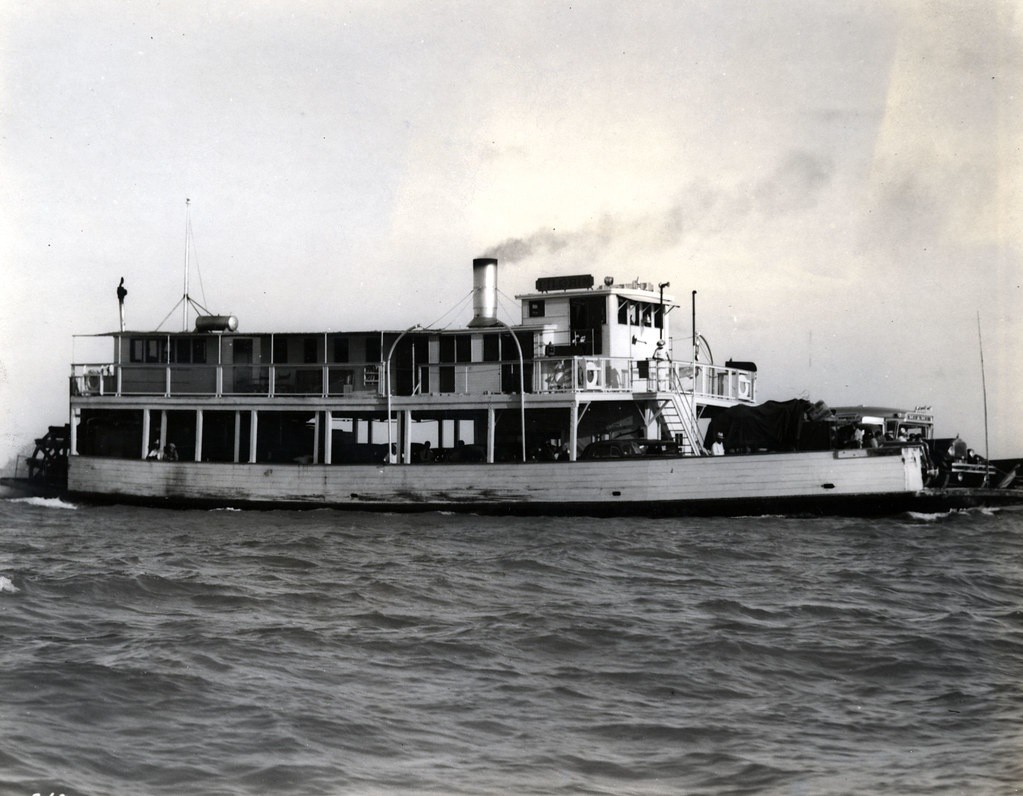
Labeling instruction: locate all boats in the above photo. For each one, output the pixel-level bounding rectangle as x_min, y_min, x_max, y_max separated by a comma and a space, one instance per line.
1, 197, 930, 524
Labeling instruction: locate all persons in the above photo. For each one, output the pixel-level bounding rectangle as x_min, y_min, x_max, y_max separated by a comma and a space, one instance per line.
711, 431, 725, 457
406, 437, 486, 468
819, 418, 1005, 486
146, 438, 162, 461
163, 443, 179, 462
